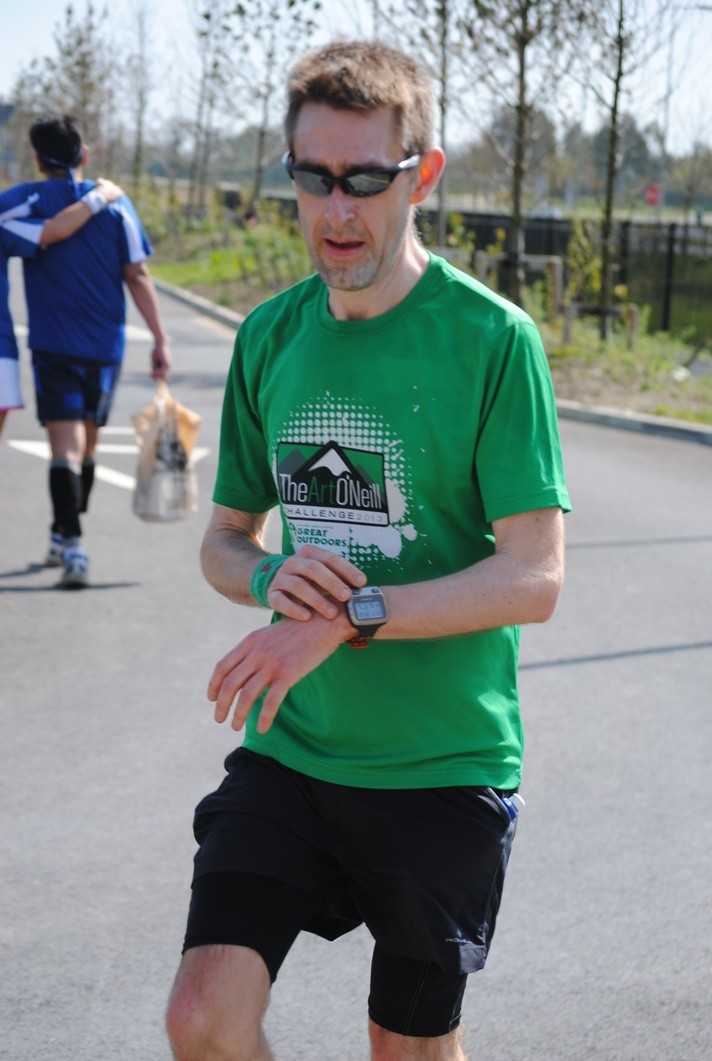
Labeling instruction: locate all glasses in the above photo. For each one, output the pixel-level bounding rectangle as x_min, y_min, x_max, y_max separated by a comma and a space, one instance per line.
281, 150, 420, 199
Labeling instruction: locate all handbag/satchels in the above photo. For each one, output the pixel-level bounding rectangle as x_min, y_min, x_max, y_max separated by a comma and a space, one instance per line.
132, 377, 198, 522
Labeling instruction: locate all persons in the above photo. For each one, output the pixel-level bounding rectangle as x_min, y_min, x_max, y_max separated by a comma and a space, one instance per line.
163, 39, 571, 1059
0, 117, 173, 588
0, 175, 124, 423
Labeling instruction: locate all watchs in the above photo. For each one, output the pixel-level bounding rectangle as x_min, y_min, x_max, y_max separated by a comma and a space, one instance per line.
344, 585, 389, 650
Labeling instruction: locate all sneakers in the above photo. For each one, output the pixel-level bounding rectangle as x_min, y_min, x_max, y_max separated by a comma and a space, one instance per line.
47, 533, 80, 565
60, 545, 88, 585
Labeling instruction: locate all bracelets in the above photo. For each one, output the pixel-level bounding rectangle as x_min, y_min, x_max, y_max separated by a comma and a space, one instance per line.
81, 189, 109, 214
249, 553, 290, 607
